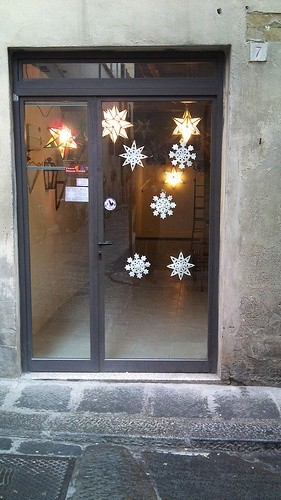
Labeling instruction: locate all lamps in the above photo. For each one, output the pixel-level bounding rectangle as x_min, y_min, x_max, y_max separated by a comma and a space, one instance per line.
101, 104, 135, 144
42, 107, 84, 160
170, 100, 202, 144
